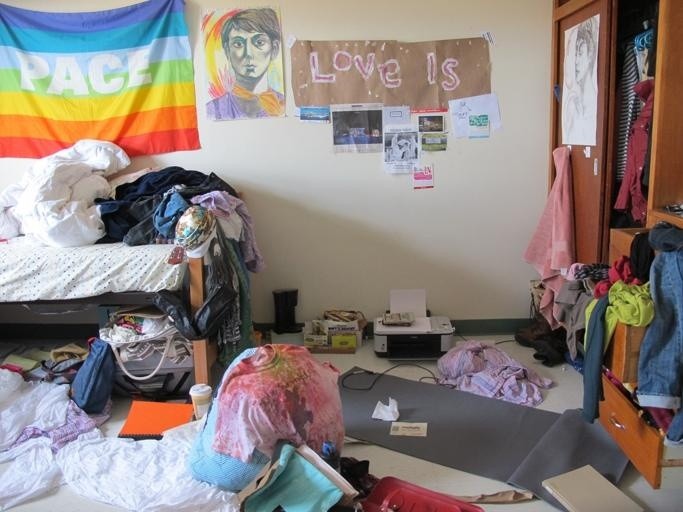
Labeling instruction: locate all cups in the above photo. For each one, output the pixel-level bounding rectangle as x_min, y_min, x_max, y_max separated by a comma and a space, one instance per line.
188, 382, 213, 421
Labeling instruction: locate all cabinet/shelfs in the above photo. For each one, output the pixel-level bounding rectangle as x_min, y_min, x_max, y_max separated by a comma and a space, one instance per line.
548, 0, 683, 489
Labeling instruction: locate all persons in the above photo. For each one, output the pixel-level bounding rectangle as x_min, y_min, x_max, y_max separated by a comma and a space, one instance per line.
562, 16, 598, 143
207, 8, 286, 120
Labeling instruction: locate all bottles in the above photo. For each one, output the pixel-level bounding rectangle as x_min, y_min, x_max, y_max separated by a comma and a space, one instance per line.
318, 441, 342, 477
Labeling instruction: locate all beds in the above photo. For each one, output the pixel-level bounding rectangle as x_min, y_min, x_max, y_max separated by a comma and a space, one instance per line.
0, 244, 220, 385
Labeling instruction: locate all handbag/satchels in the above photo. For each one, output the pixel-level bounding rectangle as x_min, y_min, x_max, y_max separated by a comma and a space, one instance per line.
69, 335, 116, 414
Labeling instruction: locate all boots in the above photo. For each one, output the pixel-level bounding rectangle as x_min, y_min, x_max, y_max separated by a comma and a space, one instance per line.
515, 318, 567, 366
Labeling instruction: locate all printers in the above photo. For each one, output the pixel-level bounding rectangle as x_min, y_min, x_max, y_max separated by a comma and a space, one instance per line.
374, 303, 456, 360
